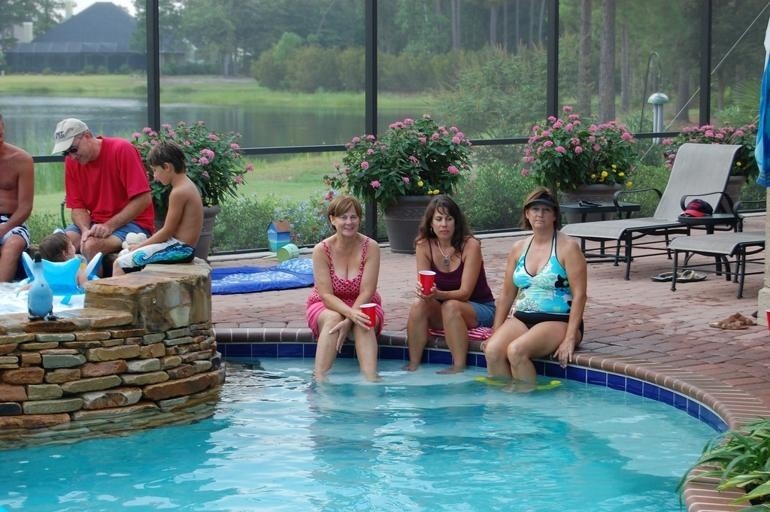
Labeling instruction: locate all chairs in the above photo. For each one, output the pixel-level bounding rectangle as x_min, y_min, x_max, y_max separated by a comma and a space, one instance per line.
559, 142, 767, 300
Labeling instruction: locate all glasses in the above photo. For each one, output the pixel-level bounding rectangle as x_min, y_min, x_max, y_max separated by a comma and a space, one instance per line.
63, 135, 83, 155
578, 200, 601, 207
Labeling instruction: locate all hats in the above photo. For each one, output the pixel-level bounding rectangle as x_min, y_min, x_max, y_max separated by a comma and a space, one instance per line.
54, 118, 88, 152
524, 198, 554, 207
680, 200, 712, 217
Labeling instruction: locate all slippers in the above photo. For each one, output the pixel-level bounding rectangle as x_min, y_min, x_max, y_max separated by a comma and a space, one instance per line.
729, 313, 756, 325
651, 272, 681, 281
709, 318, 748, 329
675, 270, 707, 281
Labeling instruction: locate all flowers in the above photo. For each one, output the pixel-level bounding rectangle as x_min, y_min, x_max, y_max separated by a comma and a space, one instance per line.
129, 117, 254, 216
319, 114, 478, 218
519, 115, 642, 192
657, 119, 762, 185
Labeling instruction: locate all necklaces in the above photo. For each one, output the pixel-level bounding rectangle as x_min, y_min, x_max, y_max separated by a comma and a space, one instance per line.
436, 242, 456, 266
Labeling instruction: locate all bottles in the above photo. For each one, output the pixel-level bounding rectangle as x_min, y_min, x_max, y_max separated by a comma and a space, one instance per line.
27, 252, 55, 319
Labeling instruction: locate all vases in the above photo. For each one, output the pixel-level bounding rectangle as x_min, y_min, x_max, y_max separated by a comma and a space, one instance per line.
721, 175, 748, 215
381, 193, 433, 256
151, 204, 222, 266
563, 182, 623, 226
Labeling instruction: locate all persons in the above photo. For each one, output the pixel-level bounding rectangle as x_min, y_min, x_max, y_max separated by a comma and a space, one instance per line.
478, 186, 588, 396
0, 113, 38, 281
302, 196, 388, 415
51, 117, 155, 280
402, 198, 496, 374
113, 142, 204, 276
13, 231, 99, 297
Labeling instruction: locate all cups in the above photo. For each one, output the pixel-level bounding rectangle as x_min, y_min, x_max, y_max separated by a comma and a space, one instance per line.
360, 304, 377, 328
418, 270, 436, 296
